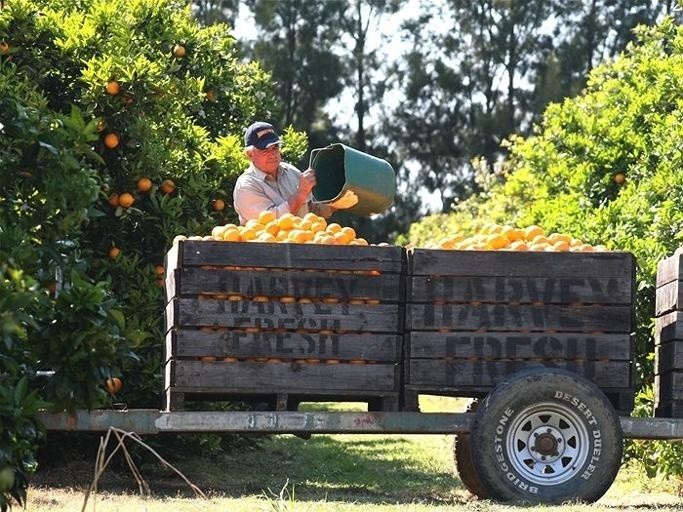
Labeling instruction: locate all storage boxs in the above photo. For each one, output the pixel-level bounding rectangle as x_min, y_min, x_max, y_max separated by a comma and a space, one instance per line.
164, 240, 404, 412
653, 254, 682, 417
404, 248, 636, 416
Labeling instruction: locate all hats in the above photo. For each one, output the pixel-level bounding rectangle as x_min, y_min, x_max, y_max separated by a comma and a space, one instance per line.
244, 121, 282, 151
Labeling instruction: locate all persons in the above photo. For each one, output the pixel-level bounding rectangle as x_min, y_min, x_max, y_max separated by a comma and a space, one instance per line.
233, 122, 333, 225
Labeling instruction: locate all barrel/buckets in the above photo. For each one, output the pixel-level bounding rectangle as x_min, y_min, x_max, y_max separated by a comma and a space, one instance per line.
309, 143, 397, 218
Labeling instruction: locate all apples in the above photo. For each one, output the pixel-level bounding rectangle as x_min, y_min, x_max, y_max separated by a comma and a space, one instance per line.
189, 209, 388, 243
106, 82, 119, 95
174, 44, 186, 57
162, 180, 174, 193
105, 377, 121, 393
109, 247, 119, 259
213, 200, 225, 211
138, 178, 151, 192
429, 221, 611, 253
615, 173, 625, 184
104, 133, 119, 148
109, 193, 133, 207
155, 265, 164, 277
